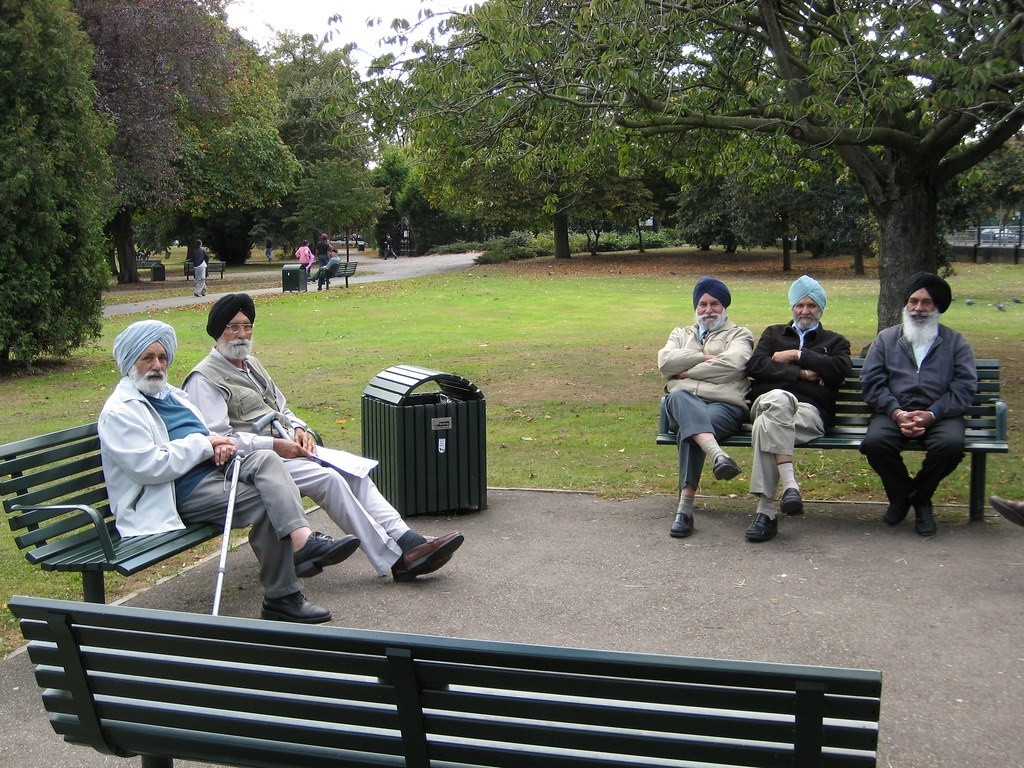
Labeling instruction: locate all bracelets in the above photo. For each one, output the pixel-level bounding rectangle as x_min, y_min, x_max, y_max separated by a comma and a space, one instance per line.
895, 411, 907, 423
805, 370, 809, 381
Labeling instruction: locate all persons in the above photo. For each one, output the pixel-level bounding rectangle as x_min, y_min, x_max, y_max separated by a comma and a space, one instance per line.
97, 319, 361, 625
989, 495, 1024, 527
180, 293, 464, 582
311, 249, 341, 290
295, 240, 311, 283
859, 272, 977, 537
265, 234, 272, 262
191, 239, 209, 297
314, 233, 330, 284
383, 234, 398, 260
657, 278, 755, 538
745, 276, 852, 542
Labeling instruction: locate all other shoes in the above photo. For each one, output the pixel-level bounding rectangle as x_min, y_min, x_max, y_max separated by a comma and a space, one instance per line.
202, 293, 205, 296
307, 278, 315, 283
194, 293, 200, 297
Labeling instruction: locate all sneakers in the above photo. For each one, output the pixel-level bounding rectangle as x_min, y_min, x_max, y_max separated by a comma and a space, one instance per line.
262, 591, 331, 625
292, 531, 361, 578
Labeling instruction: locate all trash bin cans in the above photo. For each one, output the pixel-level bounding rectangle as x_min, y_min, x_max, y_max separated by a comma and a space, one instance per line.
358, 243, 365, 252
359, 363, 487, 517
282, 263, 306, 293
151, 263, 166, 281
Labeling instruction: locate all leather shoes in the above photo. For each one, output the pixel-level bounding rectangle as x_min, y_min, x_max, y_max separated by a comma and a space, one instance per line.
745, 513, 778, 541
884, 489, 938, 536
988, 494, 1024, 527
713, 454, 743, 482
392, 531, 464, 582
669, 510, 694, 537
780, 487, 805, 517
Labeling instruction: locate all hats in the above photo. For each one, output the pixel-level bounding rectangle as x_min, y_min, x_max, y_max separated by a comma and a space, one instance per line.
321, 234, 328, 240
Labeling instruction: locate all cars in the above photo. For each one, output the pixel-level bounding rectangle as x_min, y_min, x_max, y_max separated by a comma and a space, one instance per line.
980, 228, 1020, 242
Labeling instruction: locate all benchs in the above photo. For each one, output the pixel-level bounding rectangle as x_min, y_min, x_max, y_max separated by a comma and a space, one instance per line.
136, 259, 162, 269
323, 261, 358, 290
183, 261, 227, 279
7, 593, 881, 767
0, 421, 325, 604
654, 357, 1010, 521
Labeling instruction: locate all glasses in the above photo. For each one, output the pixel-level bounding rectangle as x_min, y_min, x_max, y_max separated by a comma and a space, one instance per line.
227, 323, 253, 335
